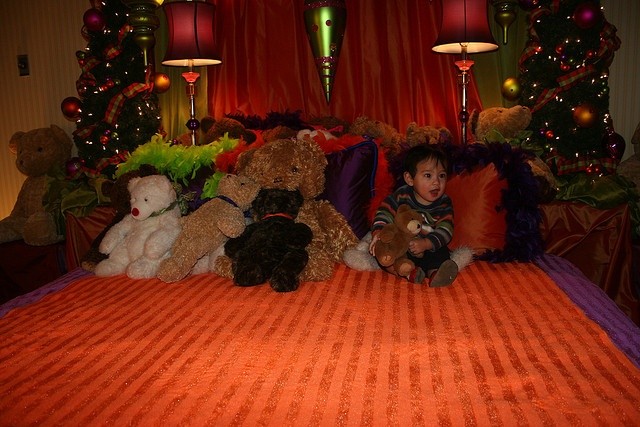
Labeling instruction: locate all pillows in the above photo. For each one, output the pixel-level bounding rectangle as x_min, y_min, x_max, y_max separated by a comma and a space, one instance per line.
318, 140, 379, 241
446, 161, 510, 258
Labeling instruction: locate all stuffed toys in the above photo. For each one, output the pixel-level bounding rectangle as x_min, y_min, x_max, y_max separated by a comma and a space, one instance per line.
468, 105, 569, 202
1, 124, 72, 246
224, 188, 313, 292
156, 174, 260, 284
94, 174, 183, 280
235, 140, 364, 282
375, 205, 425, 277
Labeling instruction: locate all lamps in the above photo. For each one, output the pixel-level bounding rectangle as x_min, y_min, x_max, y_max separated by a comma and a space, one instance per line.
433, 1, 499, 149
160, 0, 223, 144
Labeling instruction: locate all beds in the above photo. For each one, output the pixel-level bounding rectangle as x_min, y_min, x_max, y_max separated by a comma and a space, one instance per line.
2, 251, 640, 427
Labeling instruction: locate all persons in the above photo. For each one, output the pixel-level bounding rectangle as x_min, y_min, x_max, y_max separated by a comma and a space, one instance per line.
369, 146, 459, 288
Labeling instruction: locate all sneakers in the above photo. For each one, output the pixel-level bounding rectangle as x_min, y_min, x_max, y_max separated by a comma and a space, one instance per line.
409, 267, 425, 283
429, 259, 458, 287
